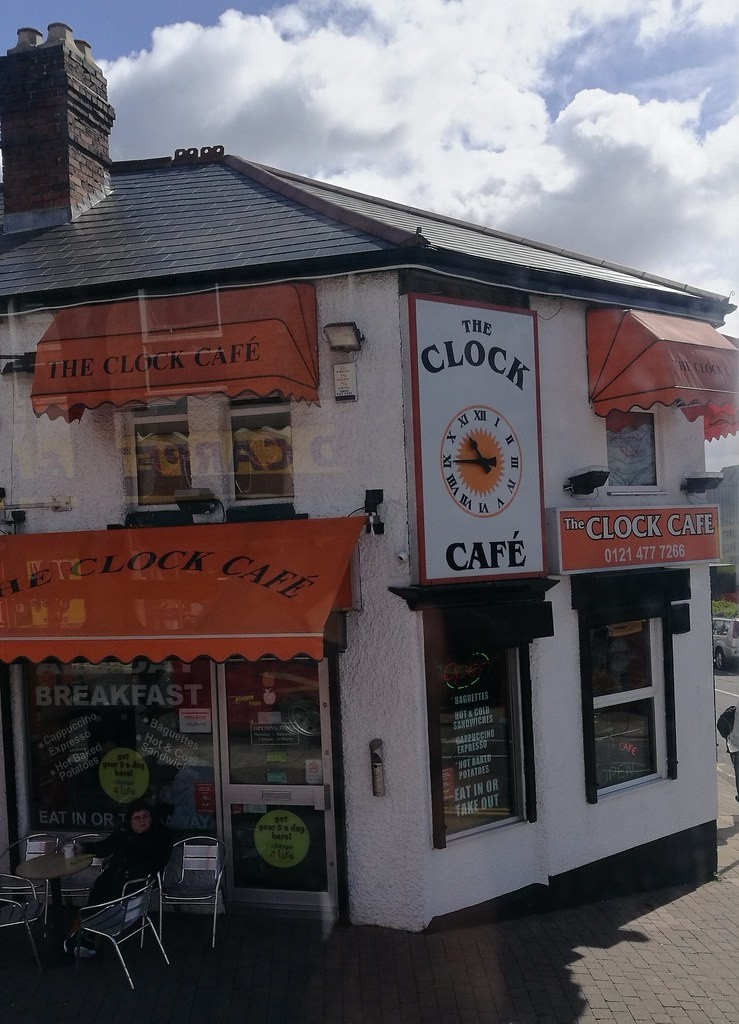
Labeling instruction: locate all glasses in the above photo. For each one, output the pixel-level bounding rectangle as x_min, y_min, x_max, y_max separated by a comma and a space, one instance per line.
131, 815, 152, 820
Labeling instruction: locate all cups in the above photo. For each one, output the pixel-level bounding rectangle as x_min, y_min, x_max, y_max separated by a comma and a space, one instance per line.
63, 844, 74, 859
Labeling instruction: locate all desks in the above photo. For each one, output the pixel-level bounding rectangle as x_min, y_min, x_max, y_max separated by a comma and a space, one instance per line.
15, 853, 93, 925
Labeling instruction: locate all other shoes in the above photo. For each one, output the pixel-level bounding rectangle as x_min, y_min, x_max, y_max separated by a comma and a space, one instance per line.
63, 928, 96, 958
735, 795, 739, 802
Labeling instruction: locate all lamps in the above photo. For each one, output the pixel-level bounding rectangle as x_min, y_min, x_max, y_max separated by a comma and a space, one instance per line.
685, 470, 724, 493
174, 488, 218, 515
324, 321, 362, 352
568, 464, 611, 495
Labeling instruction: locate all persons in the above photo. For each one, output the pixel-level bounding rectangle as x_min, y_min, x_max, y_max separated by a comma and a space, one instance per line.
64, 800, 173, 957
717, 705, 739, 802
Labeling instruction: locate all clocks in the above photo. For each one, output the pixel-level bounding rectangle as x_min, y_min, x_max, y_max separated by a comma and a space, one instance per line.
439, 403, 523, 519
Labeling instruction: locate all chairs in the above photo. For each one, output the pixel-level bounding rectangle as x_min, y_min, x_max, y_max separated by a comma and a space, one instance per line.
74, 877, 169, 992
1, 831, 60, 939
0, 873, 47, 973
51, 831, 116, 930
156, 835, 229, 948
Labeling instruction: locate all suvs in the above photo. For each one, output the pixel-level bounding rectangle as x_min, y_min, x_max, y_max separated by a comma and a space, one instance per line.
711, 618, 739, 671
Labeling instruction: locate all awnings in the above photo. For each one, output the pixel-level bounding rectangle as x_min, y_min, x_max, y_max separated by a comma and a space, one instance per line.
585, 308, 739, 443
0, 516, 369, 665
32, 284, 319, 423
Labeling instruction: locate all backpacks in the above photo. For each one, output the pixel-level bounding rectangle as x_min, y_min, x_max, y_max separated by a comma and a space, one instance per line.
717, 705, 736, 739
